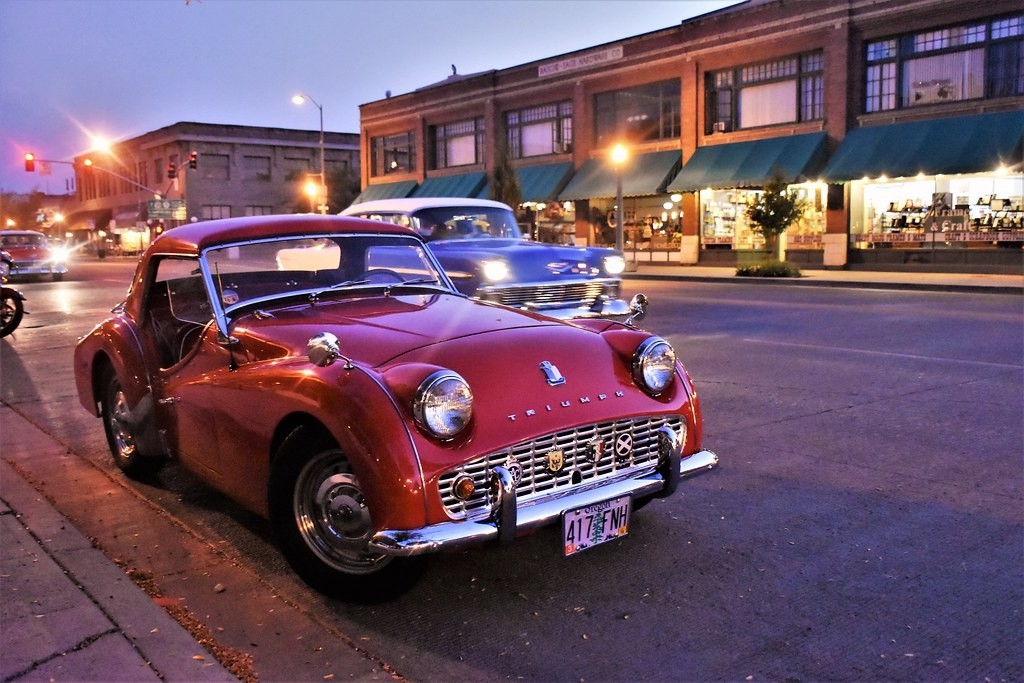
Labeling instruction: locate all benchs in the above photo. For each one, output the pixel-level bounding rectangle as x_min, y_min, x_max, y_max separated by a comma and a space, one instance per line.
155, 282, 323, 323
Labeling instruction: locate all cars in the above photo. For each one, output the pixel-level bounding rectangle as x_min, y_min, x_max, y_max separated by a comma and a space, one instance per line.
69, 213, 720, 609
1, 229, 70, 283
273, 196, 635, 328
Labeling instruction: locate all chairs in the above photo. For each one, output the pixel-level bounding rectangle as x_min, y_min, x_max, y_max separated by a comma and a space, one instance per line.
170, 322, 206, 364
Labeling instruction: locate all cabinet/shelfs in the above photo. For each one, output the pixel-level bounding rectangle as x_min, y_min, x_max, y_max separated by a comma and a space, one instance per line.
704, 189, 765, 237
881, 204, 1024, 230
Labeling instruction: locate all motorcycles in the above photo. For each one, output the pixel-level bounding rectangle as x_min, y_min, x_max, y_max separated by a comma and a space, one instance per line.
0, 235, 31, 339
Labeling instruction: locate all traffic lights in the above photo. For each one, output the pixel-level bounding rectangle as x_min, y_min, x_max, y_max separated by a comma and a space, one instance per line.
167, 162, 176, 179
154, 223, 164, 239
83, 157, 93, 177
189, 150, 198, 169
24, 152, 36, 172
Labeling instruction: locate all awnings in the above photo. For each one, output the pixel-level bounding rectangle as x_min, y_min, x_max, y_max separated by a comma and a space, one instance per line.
114, 204, 146, 227
667, 132, 829, 194
351, 179, 418, 205
63, 208, 113, 231
477, 161, 574, 202
558, 149, 681, 202
823, 108, 1024, 185
412, 172, 486, 198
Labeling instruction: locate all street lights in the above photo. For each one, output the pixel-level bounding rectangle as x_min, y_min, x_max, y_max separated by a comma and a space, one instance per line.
291, 93, 326, 215
305, 182, 319, 213
611, 144, 628, 257
95, 140, 144, 255
54, 212, 64, 237
